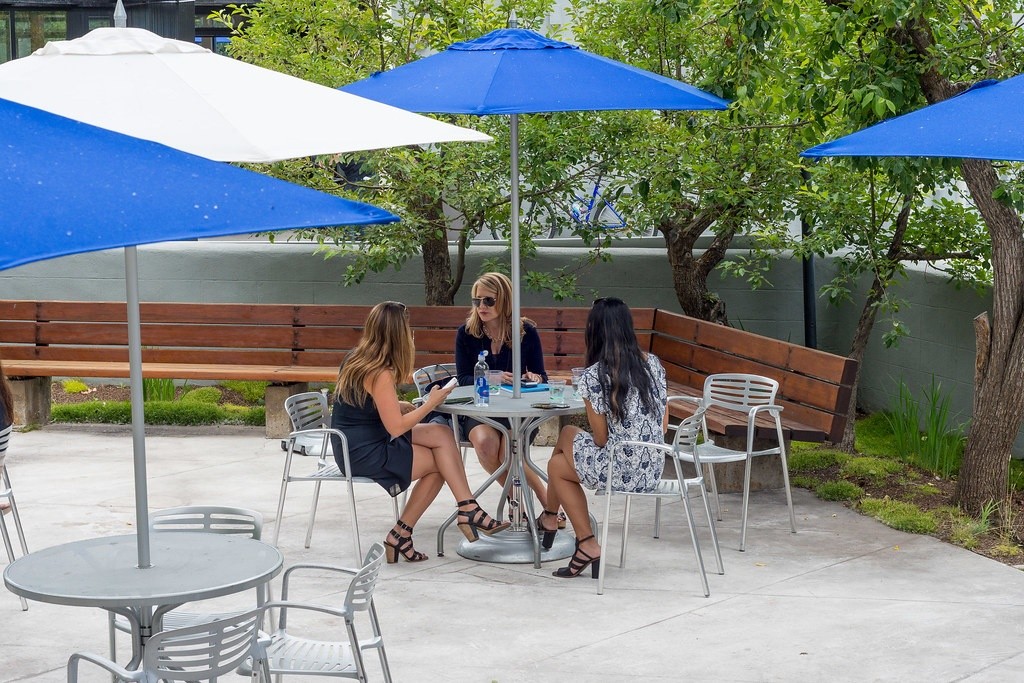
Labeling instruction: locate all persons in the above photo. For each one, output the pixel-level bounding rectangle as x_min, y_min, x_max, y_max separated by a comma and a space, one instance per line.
330, 301, 512, 564
456, 273, 567, 527
0, 365, 14, 466
527, 297, 669, 579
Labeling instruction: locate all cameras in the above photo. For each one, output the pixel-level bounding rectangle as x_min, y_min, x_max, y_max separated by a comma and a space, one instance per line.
521, 378, 537, 388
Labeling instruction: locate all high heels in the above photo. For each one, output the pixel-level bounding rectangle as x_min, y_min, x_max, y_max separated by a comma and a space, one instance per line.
552, 535, 600, 578
384, 520, 429, 563
557, 512, 566, 528
508, 495, 528, 523
456, 499, 511, 543
527, 509, 558, 548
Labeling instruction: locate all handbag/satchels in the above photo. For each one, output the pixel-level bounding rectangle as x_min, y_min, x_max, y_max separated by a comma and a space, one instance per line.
422, 376, 463, 429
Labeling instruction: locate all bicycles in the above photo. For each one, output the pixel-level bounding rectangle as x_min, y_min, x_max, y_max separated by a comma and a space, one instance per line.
491, 161, 658, 241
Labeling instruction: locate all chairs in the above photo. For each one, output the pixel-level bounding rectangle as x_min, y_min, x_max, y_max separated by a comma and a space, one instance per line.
596, 395, 724, 597
653, 371, 798, 552
0, 419, 32, 611
270, 387, 408, 550
66, 604, 272, 683
236, 542, 392, 683
148, 507, 265, 630
413, 360, 476, 471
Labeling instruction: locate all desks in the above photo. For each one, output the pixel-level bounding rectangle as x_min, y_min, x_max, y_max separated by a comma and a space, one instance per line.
423, 378, 588, 564
2, 533, 284, 683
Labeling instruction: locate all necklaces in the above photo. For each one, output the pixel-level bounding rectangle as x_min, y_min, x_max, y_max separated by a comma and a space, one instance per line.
483, 325, 501, 342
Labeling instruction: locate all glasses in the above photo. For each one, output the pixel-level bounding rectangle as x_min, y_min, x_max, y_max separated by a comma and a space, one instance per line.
390, 301, 405, 312
473, 297, 497, 307
591, 298, 602, 307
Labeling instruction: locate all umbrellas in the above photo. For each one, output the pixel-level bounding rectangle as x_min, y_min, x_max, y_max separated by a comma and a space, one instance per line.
799, 74, 1024, 162
0, 97, 401, 269
339, 9, 732, 526
0, 0, 494, 644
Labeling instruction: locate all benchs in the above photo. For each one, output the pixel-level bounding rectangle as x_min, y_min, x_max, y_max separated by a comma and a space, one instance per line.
0, 291, 858, 495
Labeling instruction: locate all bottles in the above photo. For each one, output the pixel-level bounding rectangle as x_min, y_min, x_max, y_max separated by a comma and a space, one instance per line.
473, 350, 490, 408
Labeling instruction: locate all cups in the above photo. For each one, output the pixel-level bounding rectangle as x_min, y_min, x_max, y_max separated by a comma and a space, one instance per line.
486, 370, 503, 396
571, 367, 585, 377
547, 379, 567, 404
571, 377, 584, 402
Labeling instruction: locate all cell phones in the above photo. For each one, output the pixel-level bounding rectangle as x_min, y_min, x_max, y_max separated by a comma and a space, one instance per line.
551, 404, 570, 408
442, 376, 458, 390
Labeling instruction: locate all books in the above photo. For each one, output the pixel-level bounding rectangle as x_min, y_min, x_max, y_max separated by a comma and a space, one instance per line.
501, 384, 549, 392
